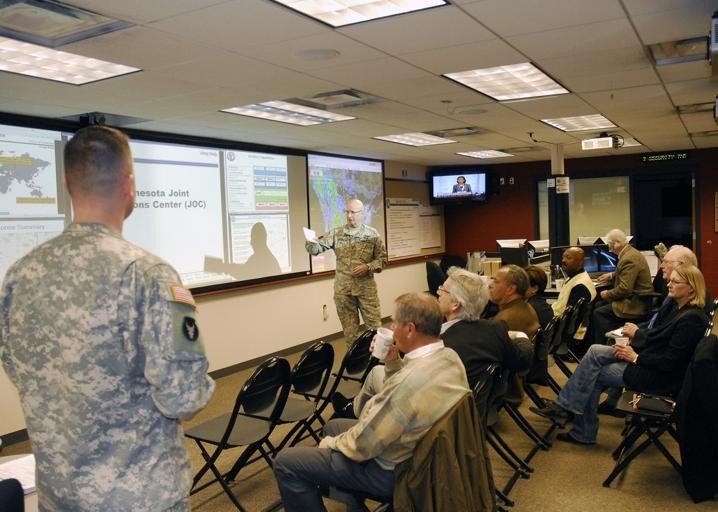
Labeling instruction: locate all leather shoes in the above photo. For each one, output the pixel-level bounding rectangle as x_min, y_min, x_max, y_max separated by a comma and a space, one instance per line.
529, 398, 616, 445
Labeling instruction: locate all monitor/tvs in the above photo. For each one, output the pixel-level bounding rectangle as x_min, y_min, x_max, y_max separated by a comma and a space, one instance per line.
570, 244, 599, 273
600, 244, 618, 271
430, 170, 488, 206
550, 246, 570, 278
496, 235, 635, 257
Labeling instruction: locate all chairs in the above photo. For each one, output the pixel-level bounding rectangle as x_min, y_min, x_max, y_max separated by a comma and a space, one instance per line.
288, 327, 386, 450
600, 277, 718, 488
182, 355, 293, 511
231, 340, 336, 482
372, 294, 588, 512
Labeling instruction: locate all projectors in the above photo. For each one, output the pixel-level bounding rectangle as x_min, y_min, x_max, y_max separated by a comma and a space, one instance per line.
580, 132, 624, 150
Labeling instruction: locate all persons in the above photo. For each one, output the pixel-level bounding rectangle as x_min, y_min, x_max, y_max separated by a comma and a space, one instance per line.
0, 125, 216, 512
332, 267, 533, 419
550, 246, 597, 341
524, 266, 554, 383
453, 176, 472, 193
530, 264, 707, 445
592, 229, 654, 344
486, 264, 540, 405
273, 289, 468, 512
306, 198, 388, 348
598, 244, 714, 414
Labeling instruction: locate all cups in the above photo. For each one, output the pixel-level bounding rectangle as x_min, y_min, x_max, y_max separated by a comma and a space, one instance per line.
371, 328, 395, 359
546, 271, 552, 289
555, 279, 564, 291
615, 337, 629, 346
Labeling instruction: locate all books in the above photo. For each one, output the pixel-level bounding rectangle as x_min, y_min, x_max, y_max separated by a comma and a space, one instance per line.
0, 453, 37, 496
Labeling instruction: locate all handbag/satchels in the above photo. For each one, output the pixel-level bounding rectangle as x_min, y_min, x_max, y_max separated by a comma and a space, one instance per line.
634, 393, 677, 413
